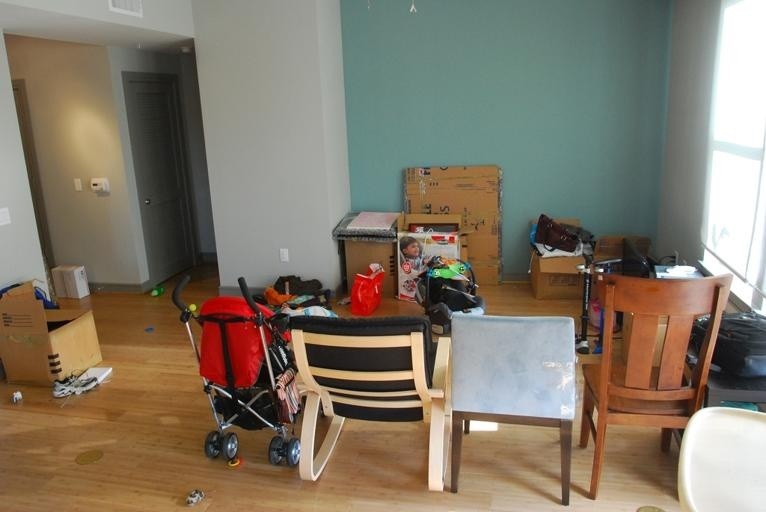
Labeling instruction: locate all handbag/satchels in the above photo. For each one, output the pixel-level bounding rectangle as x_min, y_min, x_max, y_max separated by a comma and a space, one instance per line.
350, 263, 385, 317
536, 214, 580, 251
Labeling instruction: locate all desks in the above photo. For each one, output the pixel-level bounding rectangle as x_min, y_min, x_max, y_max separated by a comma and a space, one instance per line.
678, 406, 765, 511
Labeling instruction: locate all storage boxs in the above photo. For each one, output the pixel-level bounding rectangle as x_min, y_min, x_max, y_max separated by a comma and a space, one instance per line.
0, 264, 104, 388
344, 165, 504, 302
528, 217, 661, 302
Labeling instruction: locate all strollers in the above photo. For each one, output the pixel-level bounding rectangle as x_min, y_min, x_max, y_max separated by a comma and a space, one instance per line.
169, 274, 336, 467
415, 256, 485, 335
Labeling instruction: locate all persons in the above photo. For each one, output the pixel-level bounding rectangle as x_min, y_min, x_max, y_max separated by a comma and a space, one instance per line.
399, 236, 431, 273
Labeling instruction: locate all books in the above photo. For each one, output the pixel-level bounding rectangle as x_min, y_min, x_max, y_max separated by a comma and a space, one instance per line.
78, 365, 114, 386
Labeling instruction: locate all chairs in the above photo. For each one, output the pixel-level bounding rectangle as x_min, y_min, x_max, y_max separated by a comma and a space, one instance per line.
290, 315, 451, 494
659, 317, 765, 454
578, 270, 735, 500
449, 314, 576, 507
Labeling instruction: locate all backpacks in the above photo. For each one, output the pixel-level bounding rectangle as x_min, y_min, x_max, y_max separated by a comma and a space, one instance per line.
692, 310, 766, 379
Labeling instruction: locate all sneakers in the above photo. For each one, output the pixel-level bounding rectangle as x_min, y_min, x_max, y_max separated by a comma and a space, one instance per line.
53, 374, 99, 398
